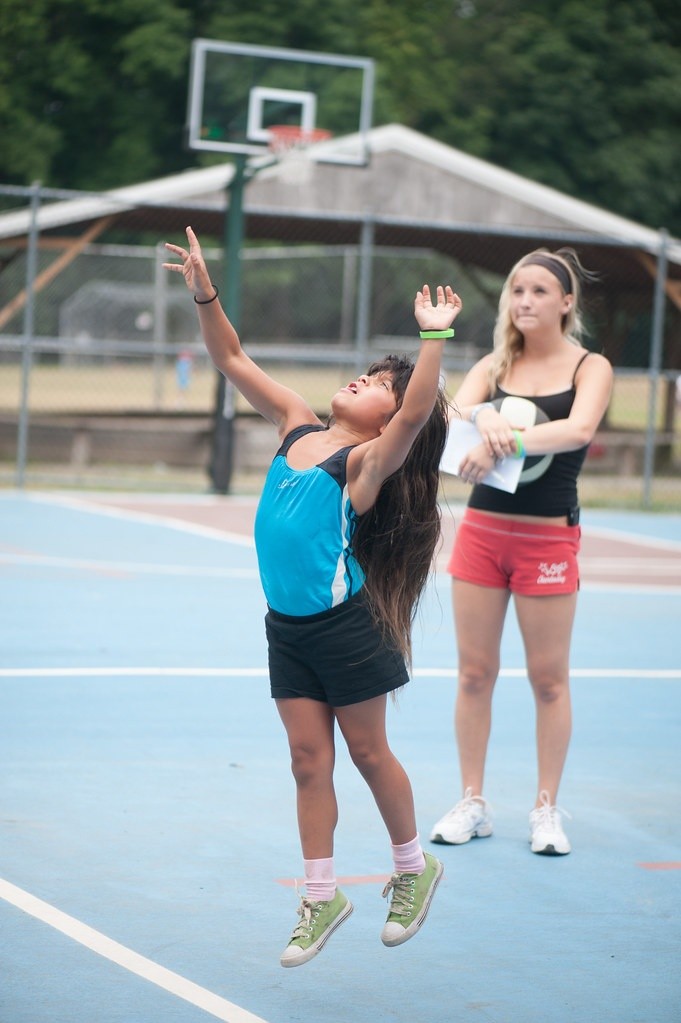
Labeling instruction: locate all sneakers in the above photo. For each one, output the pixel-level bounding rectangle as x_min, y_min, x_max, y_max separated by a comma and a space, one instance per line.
529, 790, 572, 855
381, 851, 444, 947
430, 786, 492, 844
280, 879, 354, 968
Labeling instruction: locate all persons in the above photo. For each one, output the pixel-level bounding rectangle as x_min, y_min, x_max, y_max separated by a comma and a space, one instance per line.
160, 226, 613, 968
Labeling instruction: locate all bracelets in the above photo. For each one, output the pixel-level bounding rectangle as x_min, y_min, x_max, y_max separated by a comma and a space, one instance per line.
419, 328, 455, 339
194, 285, 219, 304
513, 429, 527, 461
469, 402, 495, 424
